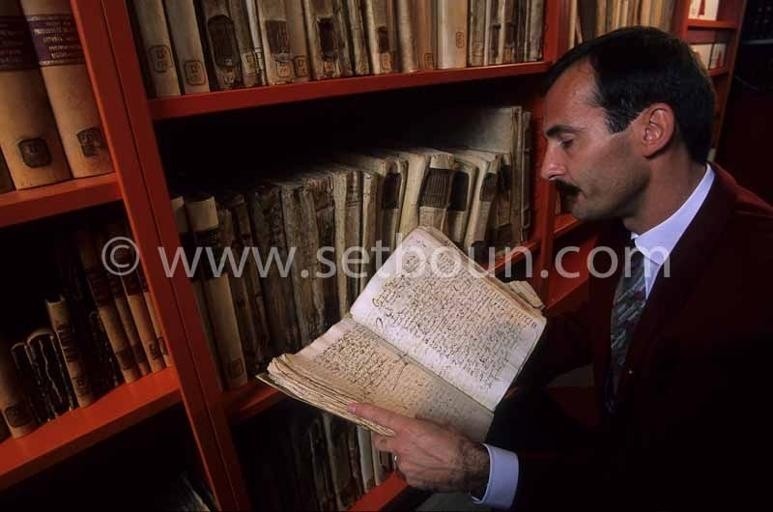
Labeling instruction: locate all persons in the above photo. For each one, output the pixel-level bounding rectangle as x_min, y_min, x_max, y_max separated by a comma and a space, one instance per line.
346, 26, 773, 512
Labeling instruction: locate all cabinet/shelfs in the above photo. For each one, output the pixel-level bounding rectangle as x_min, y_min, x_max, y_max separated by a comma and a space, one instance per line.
1, 0, 745, 512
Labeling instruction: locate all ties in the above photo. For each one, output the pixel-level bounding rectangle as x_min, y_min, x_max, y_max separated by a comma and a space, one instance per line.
609, 238, 646, 394
